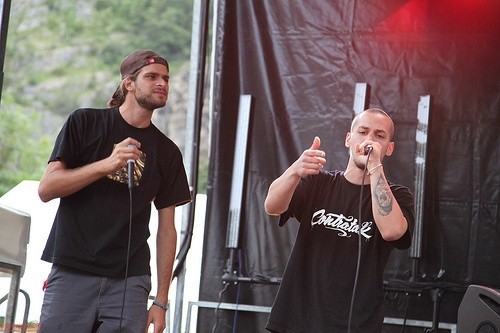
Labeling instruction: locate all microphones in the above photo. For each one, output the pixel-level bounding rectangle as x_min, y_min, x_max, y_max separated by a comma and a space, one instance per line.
127, 144, 138, 190
365, 145, 373, 155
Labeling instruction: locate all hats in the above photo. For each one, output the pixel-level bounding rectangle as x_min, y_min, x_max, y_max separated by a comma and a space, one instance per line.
112, 50, 169, 98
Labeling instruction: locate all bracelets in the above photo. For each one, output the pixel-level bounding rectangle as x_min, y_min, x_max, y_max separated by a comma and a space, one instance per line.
152, 300, 167, 311
367, 164, 383, 175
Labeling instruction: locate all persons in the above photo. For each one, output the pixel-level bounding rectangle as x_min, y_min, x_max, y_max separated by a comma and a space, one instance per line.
265, 108, 416, 333
38, 50, 191, 333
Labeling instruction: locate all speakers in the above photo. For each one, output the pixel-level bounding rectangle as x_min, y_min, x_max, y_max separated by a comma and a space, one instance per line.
456, 285, 500, 333
